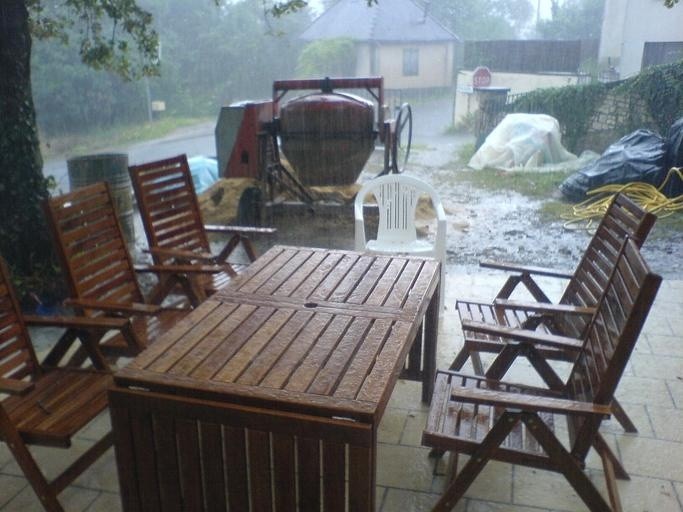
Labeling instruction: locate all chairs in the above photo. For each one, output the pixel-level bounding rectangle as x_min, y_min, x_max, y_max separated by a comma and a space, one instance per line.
0, 263, 143, 512
419, 234, 663, 512
357, 176, 448, 269
425, 190, 665, 479
42, 181, 204, 373
129, 153, 279, 310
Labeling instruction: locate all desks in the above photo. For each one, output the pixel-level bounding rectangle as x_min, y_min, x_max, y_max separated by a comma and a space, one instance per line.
105, 240, 445, 512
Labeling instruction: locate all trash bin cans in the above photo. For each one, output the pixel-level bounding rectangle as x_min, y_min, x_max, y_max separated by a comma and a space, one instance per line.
67, 153, 136, 245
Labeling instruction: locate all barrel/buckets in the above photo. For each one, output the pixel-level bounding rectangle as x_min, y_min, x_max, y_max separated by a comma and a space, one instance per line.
66, 152, 135, 243
